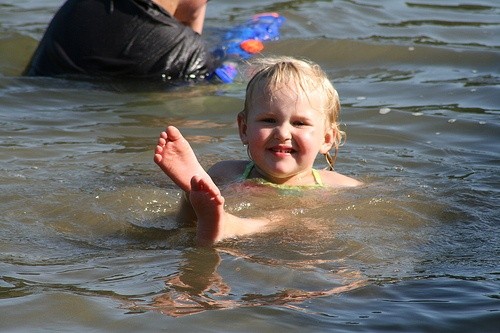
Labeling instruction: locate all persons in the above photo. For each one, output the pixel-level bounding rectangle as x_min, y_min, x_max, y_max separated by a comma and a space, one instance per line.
153, 59, 363, 245
20, 0, 221, 92
